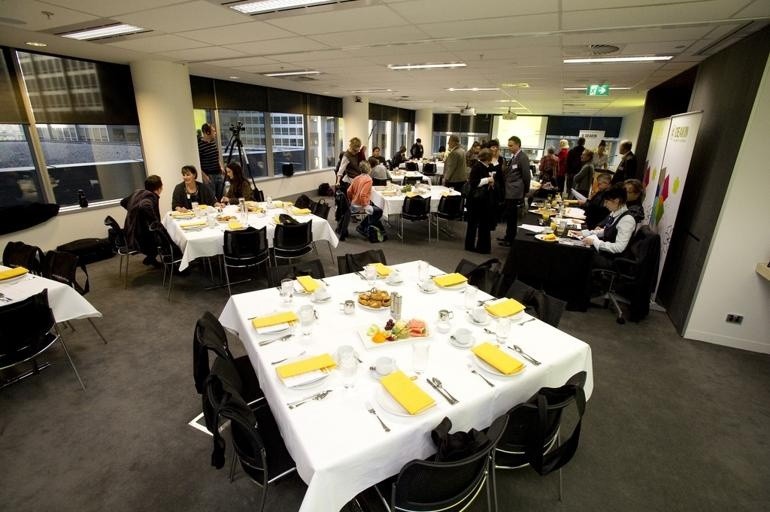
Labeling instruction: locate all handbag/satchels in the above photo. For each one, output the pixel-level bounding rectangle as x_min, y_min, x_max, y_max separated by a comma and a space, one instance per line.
467, 258, 515, 298
311, 199, 330, 220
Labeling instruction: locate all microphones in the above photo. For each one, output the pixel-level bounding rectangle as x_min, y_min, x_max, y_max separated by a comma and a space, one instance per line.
595, 155, 618, 168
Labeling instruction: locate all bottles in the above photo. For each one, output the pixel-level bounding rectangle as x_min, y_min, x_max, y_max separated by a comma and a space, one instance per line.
392, 166, 400, 176
494, 319, 510, 345
437, 309, 455, 331
389, 291, 403, 321
338, 300, 355, 315
77, 189, 88, 208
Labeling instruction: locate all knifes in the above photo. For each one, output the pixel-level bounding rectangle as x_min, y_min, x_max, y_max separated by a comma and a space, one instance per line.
467, 311, 480, 323
508, 346, 538, 366
271, 351, 306, 365
258, 335, 292, 346
425, 378, 456, 406
417, 283, 428, 292
287, 389, 333, 405
354, 271, 367, 280
313, 309, 319, 320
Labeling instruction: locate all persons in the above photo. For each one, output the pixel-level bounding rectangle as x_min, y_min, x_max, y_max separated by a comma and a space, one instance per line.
197, 123, 226, 202
538, 137, 594, 200
172, 165, 225, 212
337, 137, 386, 239
390, 145, 409, 171
221, 163, 258, 205
120, 175, 190, 277
410, 138, 423, 160
566, 140, 644, 311
439, 136, 531, 254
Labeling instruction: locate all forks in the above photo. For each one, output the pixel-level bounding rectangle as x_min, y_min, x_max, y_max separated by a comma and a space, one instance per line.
517, 318, 536, 327
366, 405, 392, 433
484, 327, 496, 335
477, 297, 497, 306
321, 279, 330, 286
466, 363, 495, 389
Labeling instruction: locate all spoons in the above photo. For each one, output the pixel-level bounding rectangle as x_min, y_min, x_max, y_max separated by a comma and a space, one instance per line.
289, 391, 328, 410
277, 286, 287, 296
514, 344, 541, 366
0, 293, 12, 303
432, 377, 460, 404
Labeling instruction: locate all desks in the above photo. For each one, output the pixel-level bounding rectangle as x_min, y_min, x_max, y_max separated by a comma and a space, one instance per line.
370, 185, 462, 235
387, 171, 433, 185
217, 259, 596, 511
504, 197, 598, 314
399, 158, 445, 176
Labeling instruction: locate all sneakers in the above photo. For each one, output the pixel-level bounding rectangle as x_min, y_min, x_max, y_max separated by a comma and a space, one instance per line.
356, 225, 368, 238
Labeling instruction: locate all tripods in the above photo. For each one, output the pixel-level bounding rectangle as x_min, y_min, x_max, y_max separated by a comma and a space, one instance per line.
218, 138, 261, 201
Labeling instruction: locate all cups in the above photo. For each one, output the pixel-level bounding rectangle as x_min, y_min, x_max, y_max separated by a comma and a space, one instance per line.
366, 265, 378, 285
336, 345, 359, 374
299, 304, 314, 336
414, 157, 439, 164
280, 278, 294, 303
536, 193, 568, 235
418, 261, 430, 281
384, 180, 455, 196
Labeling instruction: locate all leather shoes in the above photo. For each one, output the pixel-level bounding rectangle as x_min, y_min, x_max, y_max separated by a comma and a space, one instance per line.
496, 237, 507, 243
499, 241, 512, 247
142, 258, 162, 270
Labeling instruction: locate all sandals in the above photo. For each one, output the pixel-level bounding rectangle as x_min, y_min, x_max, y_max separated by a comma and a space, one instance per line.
369, 220, 388, 243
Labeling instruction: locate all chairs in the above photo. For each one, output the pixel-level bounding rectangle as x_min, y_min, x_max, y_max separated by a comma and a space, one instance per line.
336, 158, 660, 325
104, 190, 339, 301
454, 258, 503, 297
337, 250, 388, 276
273, 259, 326, 286
490, 368, 587, 510
355, 414, 509, 512
193, 306, 298, 512
0, 241, 107, 393
505, 279, 566, 329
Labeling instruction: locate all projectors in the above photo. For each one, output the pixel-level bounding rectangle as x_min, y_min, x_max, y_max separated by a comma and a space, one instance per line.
503, 114, 517, 120
461, 107, 476, 116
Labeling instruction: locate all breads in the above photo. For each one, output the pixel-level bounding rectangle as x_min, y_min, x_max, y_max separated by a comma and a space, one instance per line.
216, 215, 235, 222
359, 289, 391, 308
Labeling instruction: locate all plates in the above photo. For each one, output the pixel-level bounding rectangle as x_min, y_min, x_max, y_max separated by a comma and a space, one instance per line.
360, 327, 430, 348
420, 285, 437, 294
284, 358, 330, 391
533, 234, 559, 244
435, 274, 468, 289
484, 297, 525, 320
377, 386, 431, 418
0, 275, 25, 283
172, 195, 309, 231
449, 328, 476, 349
469, 314, 492, 326
296, 280, 331, 305
384, 276, 404, 287
474, 353, 525, 378
358, 302, 390, 311
